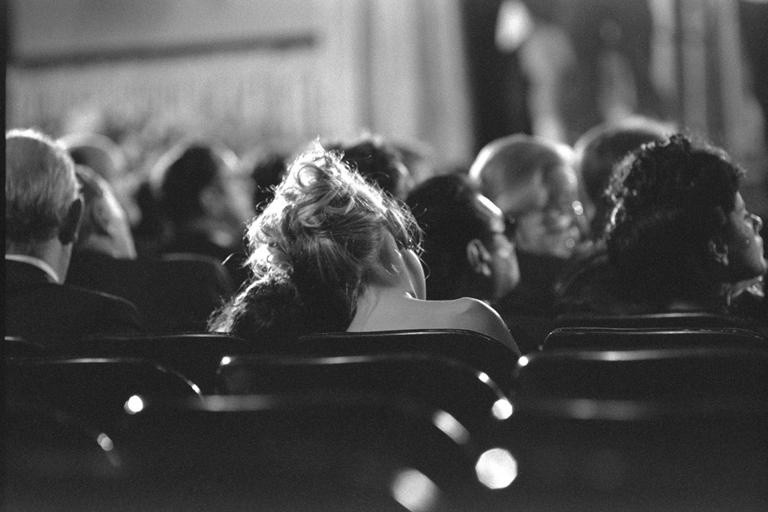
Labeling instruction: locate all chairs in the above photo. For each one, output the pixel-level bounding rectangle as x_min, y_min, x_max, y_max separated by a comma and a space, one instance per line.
0, 249, 768, 512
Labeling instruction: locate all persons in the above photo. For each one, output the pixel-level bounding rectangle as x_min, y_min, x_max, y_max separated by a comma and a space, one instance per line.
2, 112, 768, 353
